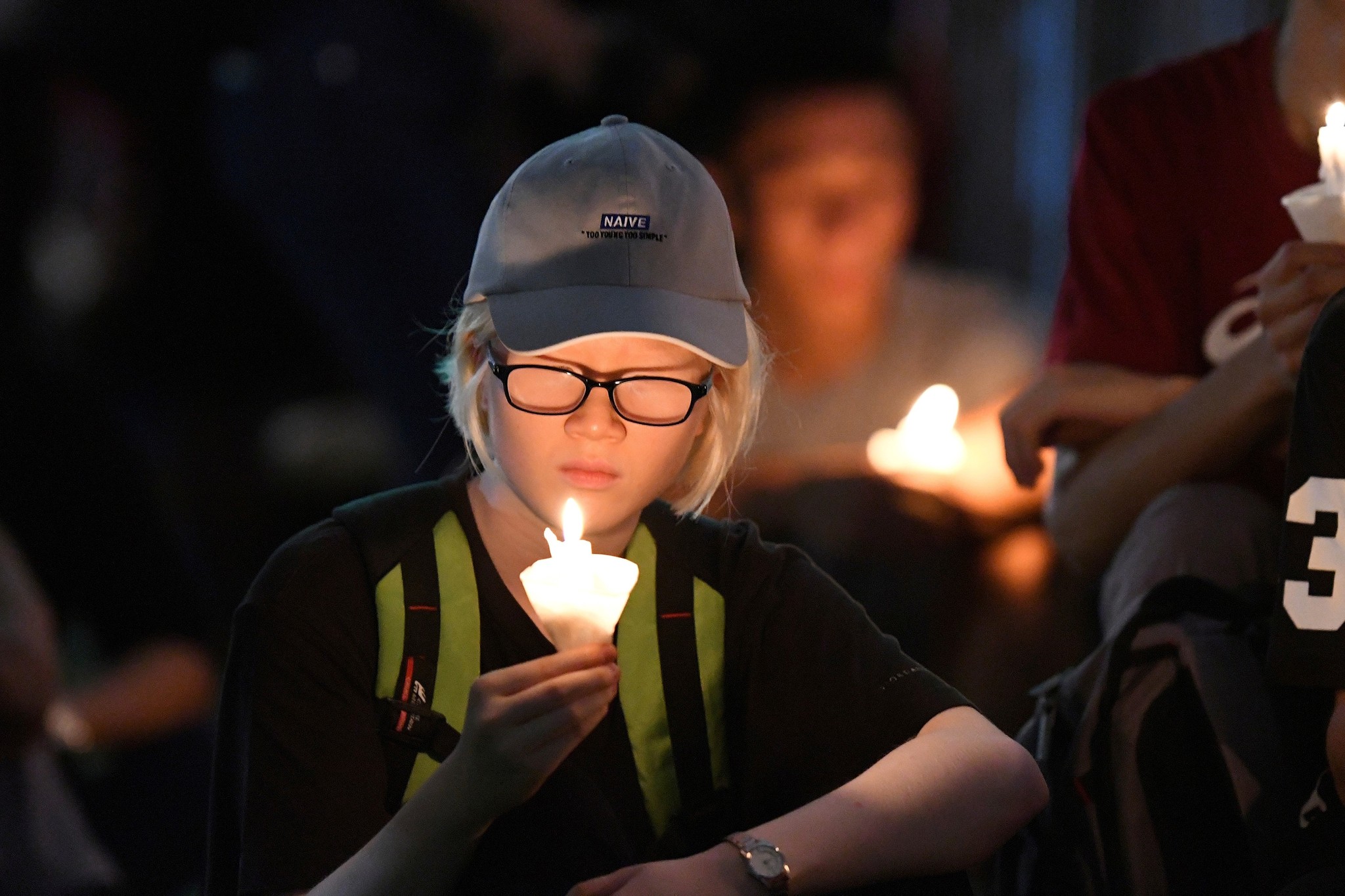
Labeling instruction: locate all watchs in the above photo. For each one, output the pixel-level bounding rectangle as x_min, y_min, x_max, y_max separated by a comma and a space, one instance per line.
725, 832, 792, 896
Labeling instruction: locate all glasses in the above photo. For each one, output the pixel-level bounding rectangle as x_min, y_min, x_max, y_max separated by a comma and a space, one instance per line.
479, 334, 718, 427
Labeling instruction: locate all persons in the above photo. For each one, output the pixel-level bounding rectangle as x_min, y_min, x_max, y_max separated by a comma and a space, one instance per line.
410, 0, 1069, 517
210, 115, 1046, 896
0, 80, 221, 896
1000, 0, 1345, 896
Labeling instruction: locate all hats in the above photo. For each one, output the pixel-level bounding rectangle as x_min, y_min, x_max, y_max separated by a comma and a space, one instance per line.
461, 115, 755, 370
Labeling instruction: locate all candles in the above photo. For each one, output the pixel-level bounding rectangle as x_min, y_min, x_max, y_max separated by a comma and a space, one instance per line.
545, 497, 593, 571
